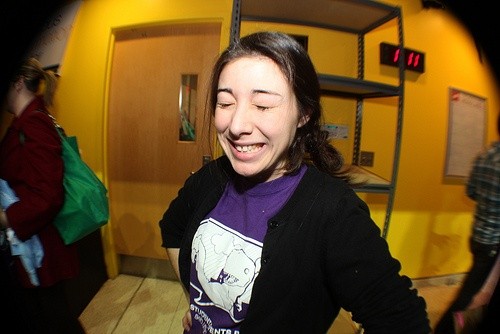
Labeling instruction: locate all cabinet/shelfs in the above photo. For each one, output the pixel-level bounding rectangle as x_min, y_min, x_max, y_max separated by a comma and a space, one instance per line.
230, 0, 405, 242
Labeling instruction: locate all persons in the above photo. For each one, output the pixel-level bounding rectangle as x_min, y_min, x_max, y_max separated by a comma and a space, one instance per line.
434, 112, 500, 334
159, 31, 433, 334
0, 58, 86, 334
